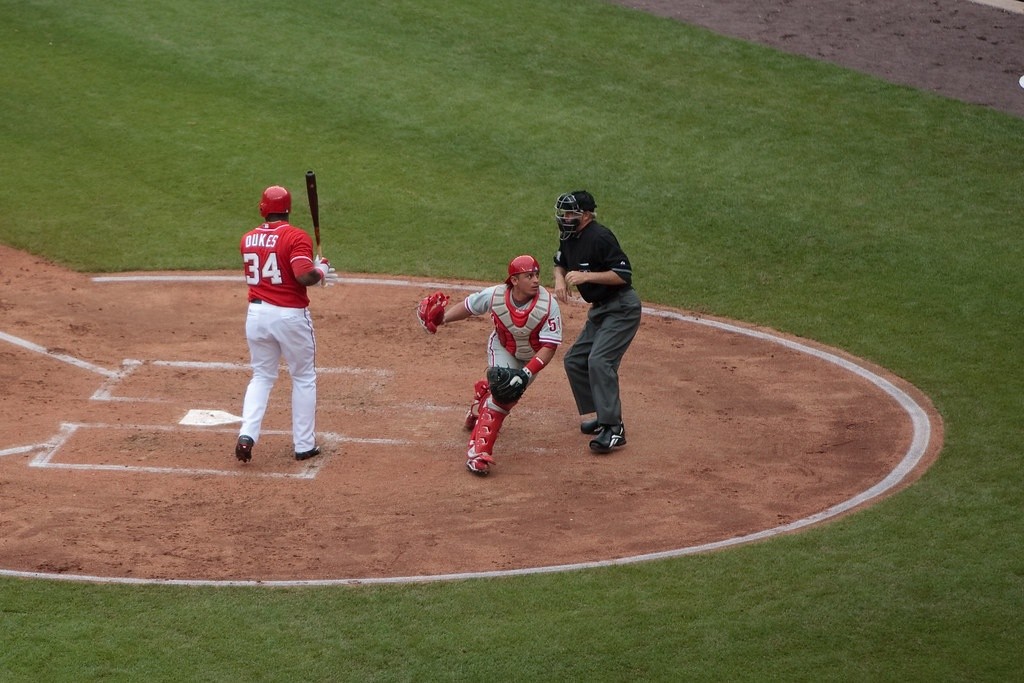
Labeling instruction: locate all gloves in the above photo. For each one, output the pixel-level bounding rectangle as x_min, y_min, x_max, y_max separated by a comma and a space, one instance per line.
313, 268, 338, 288
314, 254, 330, 276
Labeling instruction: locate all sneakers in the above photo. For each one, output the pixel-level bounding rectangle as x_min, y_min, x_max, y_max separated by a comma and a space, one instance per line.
466, 411, 476, 430
581, 419, 604, 434
590, 422, 626, 452
235, 436, 254, 463
467, 456, 491, 474
295, 447, 320, 460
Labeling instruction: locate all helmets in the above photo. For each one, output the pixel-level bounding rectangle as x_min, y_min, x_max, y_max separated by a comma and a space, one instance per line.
259, 185, 292, 218
504, 255, 540, 285
571, 190, 596, 211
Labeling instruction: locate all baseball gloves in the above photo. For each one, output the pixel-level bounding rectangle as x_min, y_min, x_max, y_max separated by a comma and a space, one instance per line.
487, 367, 529, 404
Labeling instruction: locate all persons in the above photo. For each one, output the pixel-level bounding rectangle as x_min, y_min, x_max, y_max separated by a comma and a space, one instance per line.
417, 255, 563, 474
553, 191, 642, 454
234, 186, 339, 463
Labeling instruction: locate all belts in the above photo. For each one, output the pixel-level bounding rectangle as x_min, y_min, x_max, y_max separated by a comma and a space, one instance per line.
250, 298, 261, 304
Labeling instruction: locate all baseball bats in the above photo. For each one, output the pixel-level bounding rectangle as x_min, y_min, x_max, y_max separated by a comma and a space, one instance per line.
306, 169, 328, 288
